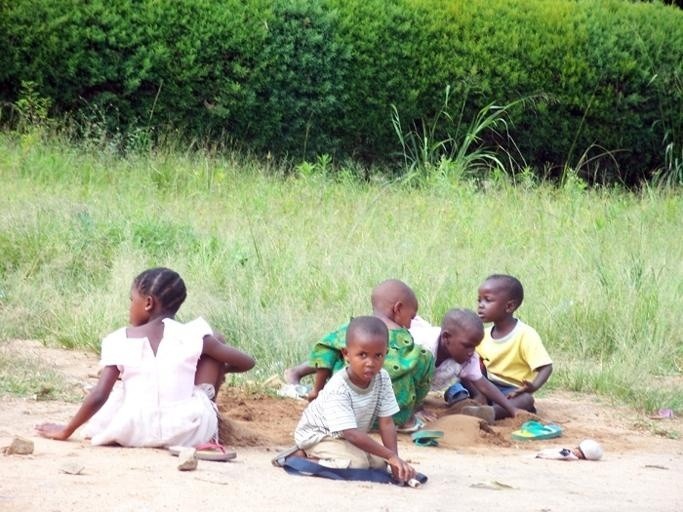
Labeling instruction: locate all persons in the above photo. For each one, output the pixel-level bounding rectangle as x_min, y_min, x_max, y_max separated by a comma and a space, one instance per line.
445, 274, 553, 422
299, 279, 435, 430
35, 268, 254, 450
272, 315, 415, 482
284, 307, 524, 416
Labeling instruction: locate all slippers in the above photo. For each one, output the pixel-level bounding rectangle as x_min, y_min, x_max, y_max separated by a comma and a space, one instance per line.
397, 418, 423, 433
512, 421, 561, 440
462, 405, 495, 422
412, 431, 444, 448
169, 443, 235, 461
444, 385, 469, 405
271, 446, 302, 468
